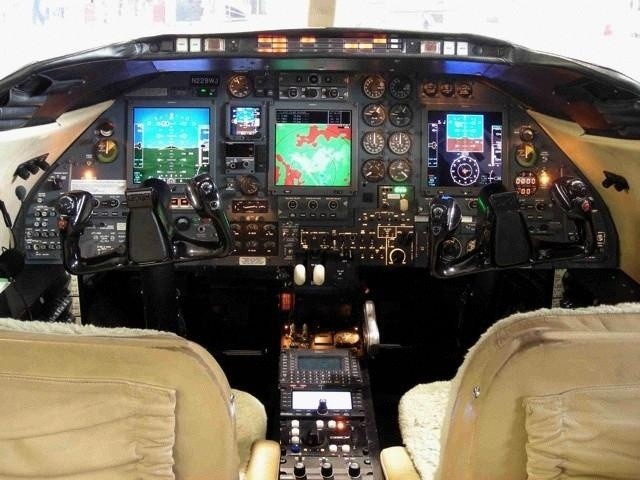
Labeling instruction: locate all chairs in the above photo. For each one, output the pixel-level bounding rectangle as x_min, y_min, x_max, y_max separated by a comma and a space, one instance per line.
0, 314, 283, 480
374, 300, 640, 480
